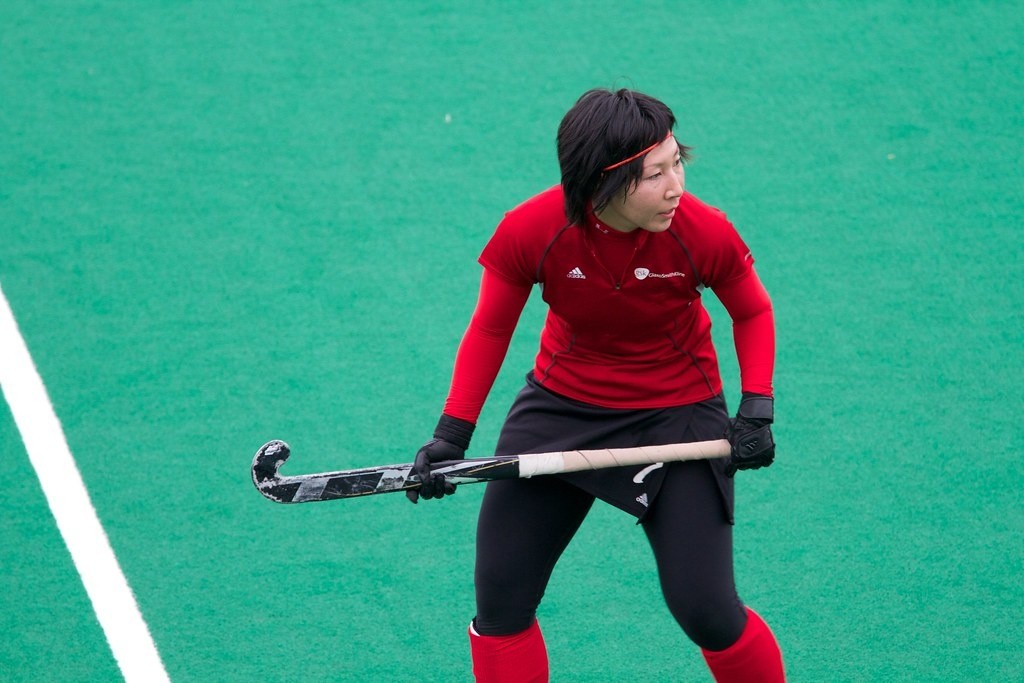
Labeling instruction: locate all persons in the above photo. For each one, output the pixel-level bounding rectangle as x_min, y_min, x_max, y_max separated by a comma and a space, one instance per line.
405, 90, 788, 682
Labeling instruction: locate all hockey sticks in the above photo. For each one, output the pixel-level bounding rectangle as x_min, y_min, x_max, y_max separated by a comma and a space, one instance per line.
246, 435, 740, 507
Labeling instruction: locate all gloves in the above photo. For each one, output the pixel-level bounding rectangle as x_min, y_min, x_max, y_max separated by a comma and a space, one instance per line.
406, 415, 476, 504
724, 392, 776, 478
